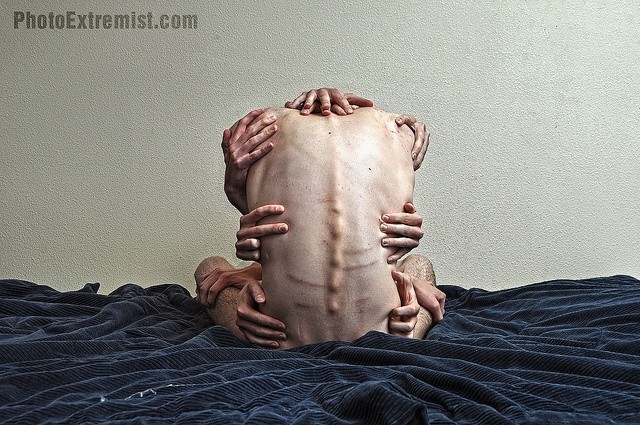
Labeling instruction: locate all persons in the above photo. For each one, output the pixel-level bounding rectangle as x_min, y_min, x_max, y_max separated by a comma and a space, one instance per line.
192, 85, 449, 351
